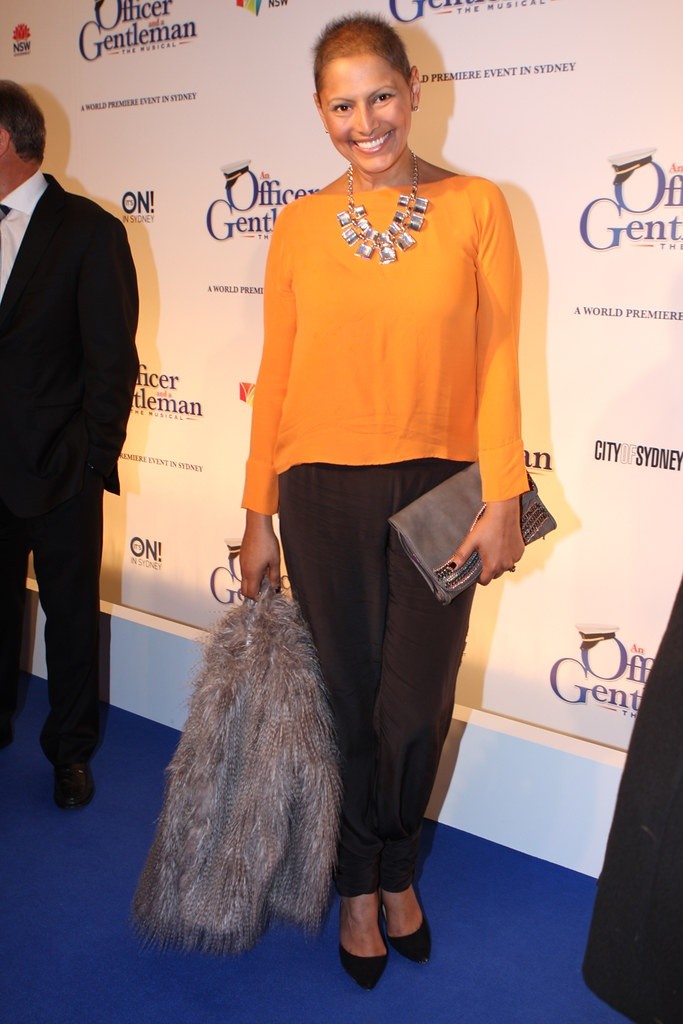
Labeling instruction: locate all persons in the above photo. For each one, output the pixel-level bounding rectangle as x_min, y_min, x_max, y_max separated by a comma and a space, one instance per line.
0, 81, 140, 807
237, 15, 528, 991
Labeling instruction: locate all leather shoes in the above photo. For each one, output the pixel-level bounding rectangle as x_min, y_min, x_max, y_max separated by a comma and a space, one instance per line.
54, 759, 94, 810
0, 731, 11, 748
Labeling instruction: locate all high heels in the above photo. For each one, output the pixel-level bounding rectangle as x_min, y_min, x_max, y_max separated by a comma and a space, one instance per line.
338, 883, 389, 992
380, 882, 431, 964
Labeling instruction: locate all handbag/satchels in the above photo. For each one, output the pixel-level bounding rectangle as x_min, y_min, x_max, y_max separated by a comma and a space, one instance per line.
386, 461, 557, 607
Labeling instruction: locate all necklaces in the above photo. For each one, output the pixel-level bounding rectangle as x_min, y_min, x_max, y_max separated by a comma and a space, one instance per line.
338, 150, 428, 265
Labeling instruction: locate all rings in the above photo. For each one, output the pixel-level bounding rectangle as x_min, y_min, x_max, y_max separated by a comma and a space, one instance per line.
509, 566, 516, 573
